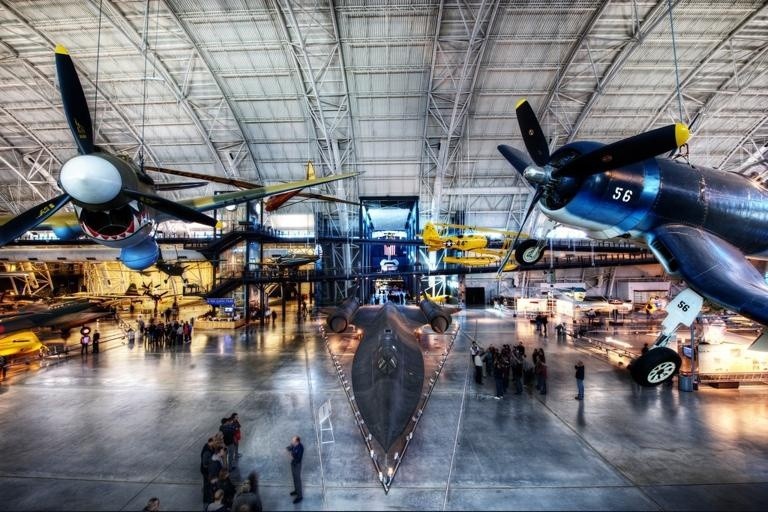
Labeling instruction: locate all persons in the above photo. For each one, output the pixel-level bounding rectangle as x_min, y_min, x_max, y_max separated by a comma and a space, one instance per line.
467, 338, 558, 399
79, 332, 90, 357
535, 311, 541, 331
554, 308, 604, 339
91, 328, 101, 354
124, 297, 199, 348
265, 307, 271, 325
139, 497, 163, 512
284, 434, 305, 503
0, 354, 8, 381
574, 359, 586, 400
541, 315, 549, 337
196, 412, 263, 512
272, 310, 277, 326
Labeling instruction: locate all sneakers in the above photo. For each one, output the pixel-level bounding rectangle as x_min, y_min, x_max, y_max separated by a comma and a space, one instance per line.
290, 490, 303, 504
475, 374, 585, 400
